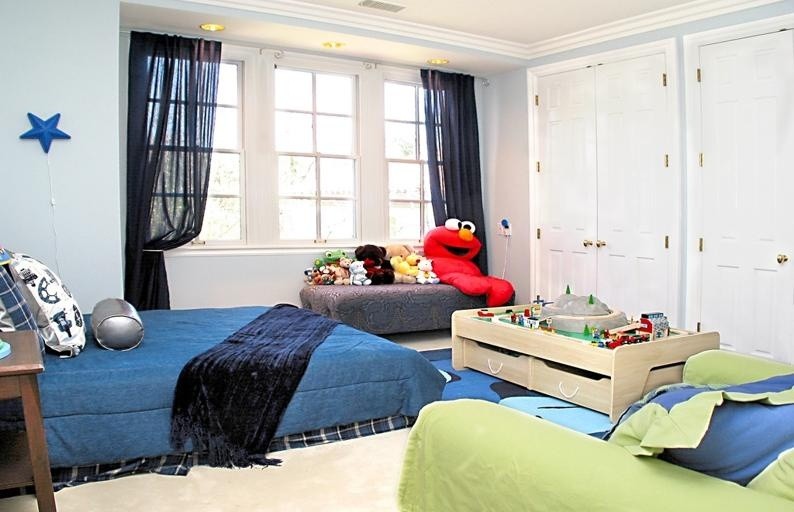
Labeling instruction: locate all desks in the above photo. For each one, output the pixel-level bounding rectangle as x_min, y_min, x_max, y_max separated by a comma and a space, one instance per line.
0, 331, 57, 512
451, 303, 720, 424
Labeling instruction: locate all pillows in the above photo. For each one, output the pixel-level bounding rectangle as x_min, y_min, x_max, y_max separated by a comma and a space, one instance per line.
91, 298, 144, 352
0, 248, 47, 358
8, 253, 87, 359
604, 373, 794, 486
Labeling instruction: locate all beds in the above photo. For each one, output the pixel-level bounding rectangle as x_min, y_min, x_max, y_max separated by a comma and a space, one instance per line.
0, 249, 446, 499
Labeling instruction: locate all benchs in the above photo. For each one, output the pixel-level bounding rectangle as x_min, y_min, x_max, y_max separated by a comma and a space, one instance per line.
300, 284, 515, 336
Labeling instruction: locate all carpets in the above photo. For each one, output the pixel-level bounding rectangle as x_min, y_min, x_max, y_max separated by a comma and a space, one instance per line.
418, 348, 611, 439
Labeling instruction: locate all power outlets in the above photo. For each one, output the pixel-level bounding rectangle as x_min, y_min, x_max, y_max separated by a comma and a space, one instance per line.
496, 223, 512, 236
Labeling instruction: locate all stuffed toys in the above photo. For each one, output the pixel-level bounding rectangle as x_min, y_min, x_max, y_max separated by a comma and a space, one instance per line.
422, 218, 514, 308
303, 245, 439, 287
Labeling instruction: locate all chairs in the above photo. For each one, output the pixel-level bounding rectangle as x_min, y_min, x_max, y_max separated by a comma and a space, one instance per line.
400, 349, 794, 512
